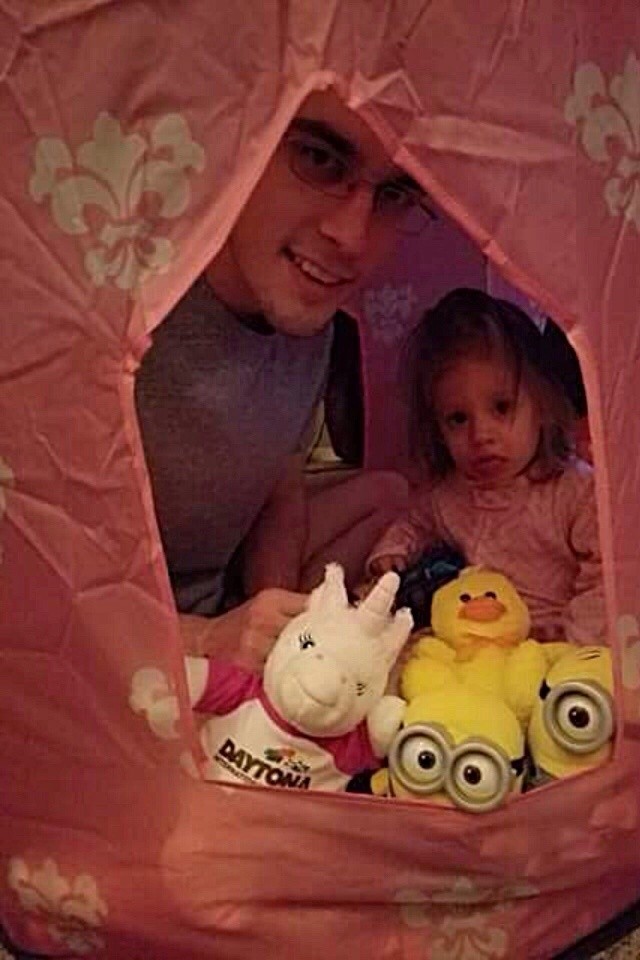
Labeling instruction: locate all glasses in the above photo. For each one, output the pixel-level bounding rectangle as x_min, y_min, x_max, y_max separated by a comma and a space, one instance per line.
282, 136, 435, 234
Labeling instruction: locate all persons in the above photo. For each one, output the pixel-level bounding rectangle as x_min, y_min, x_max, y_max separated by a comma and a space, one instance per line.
133, 84, 437, 674
364, 288, 607, 646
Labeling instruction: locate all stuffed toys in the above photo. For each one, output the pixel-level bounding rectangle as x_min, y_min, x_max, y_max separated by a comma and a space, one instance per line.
128, 560, 616, 931
